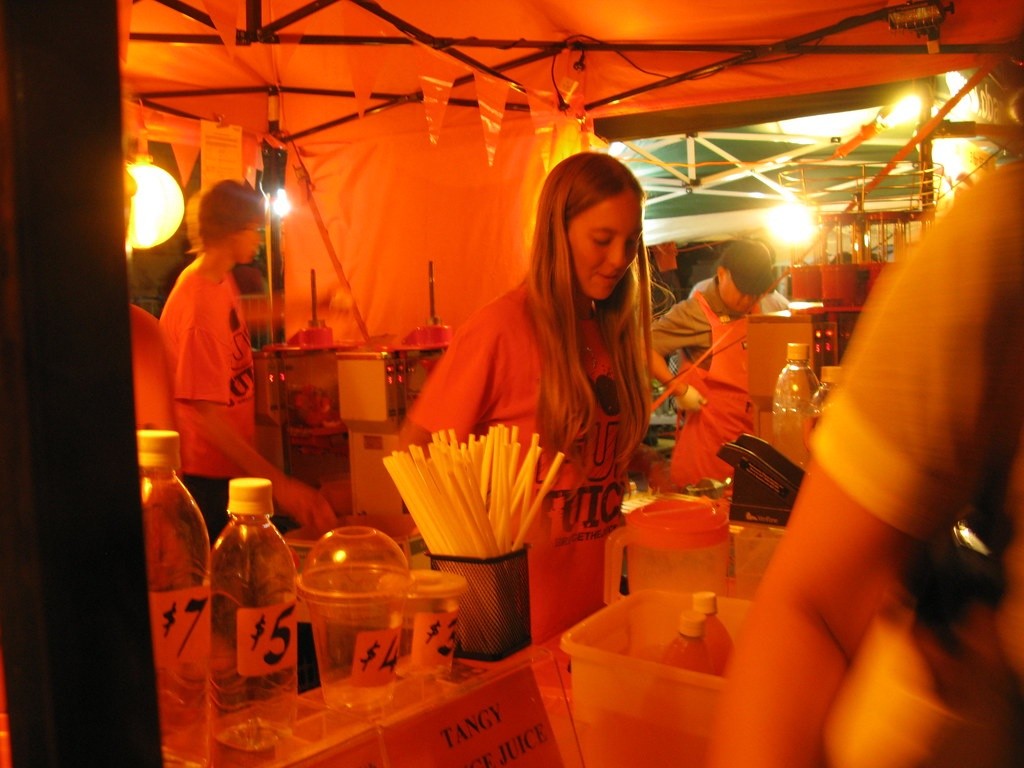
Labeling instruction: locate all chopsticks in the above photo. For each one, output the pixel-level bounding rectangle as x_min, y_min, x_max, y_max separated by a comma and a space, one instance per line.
380, 420, 565, 559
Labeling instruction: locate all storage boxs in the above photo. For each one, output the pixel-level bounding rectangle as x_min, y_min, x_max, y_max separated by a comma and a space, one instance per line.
559, 590, 753, 732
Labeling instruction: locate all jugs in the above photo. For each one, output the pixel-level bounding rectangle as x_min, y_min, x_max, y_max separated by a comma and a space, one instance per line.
601, 497, 731, 606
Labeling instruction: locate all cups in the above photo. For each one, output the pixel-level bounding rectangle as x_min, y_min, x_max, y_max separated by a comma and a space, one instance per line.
391, 567, 470, 681
296, 561, 408, 714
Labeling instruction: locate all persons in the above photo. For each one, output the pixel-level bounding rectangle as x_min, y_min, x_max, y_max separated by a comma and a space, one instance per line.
644, 237, 775, 486
689, 229, 792, 315
160, 178, 337, 554
130, 302, 179, 508
715, 160, 1024, 768
832, 252, 851, 265
397, 151, 653, 642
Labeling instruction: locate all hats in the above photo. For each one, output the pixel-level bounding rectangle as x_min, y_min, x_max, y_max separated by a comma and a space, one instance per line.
198, 178, 266, 244
719, 240, 775, 295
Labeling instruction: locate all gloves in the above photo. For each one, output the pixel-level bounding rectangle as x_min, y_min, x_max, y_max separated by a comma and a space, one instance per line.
673, 383, 707, 411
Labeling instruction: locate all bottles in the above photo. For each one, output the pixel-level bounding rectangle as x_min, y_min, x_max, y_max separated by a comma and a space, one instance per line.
136, 428, 212, 767
770, 342, 843, 472
664, 590, 735, 677
205, 475, 298, 753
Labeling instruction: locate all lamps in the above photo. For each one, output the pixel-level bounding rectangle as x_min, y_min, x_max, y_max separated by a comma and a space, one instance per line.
126, 98, 185, 249
